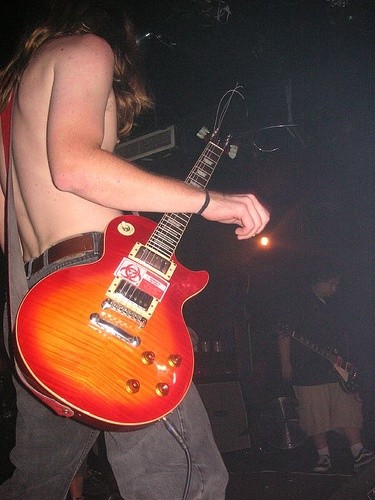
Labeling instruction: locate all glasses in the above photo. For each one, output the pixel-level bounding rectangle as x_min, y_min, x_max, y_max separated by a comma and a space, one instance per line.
133, 27, 179, 48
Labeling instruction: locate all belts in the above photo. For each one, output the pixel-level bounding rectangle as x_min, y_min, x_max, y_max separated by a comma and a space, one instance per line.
23, 231, 105, 277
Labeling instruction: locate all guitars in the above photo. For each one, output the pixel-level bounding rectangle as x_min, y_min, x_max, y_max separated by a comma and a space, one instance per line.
271, 322, 366, 394
12, 126, 239, 433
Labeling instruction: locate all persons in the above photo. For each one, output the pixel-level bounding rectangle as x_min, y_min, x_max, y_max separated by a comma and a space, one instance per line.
0, 0, 272, 500
277, 262, 375, 473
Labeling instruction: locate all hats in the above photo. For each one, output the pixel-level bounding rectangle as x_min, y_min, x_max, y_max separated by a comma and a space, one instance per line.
118, 0, 178, 42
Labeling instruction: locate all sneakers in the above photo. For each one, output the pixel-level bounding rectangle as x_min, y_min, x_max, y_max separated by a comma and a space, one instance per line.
312, 454, 337, 472
352, 447, 375, 472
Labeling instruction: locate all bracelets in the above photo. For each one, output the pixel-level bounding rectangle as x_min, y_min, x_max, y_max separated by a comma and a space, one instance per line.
196, 188, 211, 217
